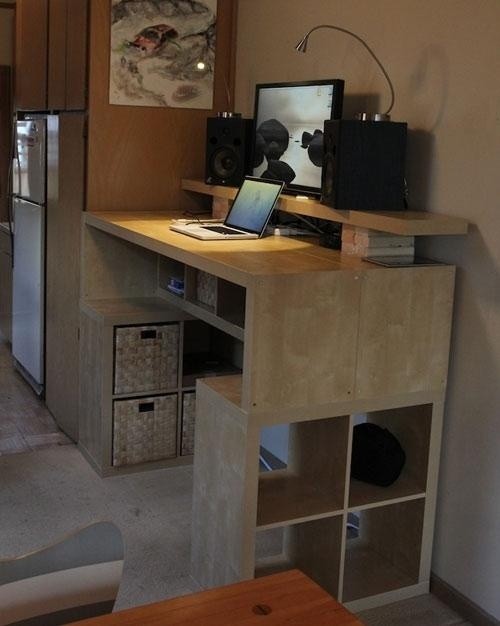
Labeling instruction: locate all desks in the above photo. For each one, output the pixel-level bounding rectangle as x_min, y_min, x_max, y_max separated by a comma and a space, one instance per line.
62, 566, 368, 625
79, 176, 470, 610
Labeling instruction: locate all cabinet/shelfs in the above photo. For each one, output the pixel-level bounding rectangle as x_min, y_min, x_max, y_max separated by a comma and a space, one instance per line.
14, 0, 90, 109
44, 110, 235, 446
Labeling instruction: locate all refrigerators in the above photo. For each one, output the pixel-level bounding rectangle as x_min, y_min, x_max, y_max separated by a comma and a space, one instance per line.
7, 112, 46, 398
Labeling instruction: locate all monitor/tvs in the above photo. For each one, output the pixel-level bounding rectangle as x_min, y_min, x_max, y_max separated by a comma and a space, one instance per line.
245, 79, 345, 199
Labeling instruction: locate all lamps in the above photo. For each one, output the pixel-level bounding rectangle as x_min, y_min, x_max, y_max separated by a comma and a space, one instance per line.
297, 23, 398, 120
196, 57, 243, 117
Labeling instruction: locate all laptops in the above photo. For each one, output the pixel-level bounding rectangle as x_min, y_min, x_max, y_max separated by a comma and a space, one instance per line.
169, 174, 285, 240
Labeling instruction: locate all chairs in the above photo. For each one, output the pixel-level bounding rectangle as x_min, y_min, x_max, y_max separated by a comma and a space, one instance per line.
0, 517, 124, 624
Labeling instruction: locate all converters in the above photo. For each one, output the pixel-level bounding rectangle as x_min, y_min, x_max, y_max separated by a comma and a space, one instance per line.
266, 225, 297, 236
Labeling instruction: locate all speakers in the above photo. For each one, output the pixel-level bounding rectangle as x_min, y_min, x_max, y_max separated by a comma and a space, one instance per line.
204, 111, 253, 187
320, 112, 407, 211
